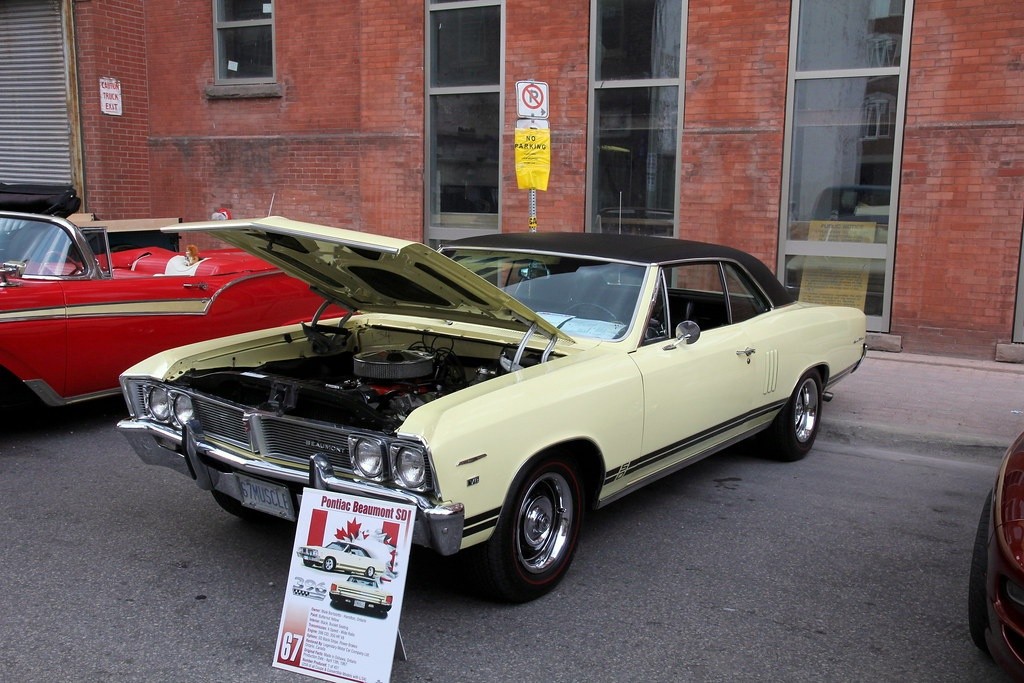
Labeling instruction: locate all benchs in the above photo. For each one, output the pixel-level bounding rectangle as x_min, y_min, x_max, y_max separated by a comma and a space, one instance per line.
563, 284, 755, 332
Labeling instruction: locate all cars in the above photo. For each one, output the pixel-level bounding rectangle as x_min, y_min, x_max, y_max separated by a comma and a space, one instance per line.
298, 540, 386, 578
966, 433, 1024, 683
114, 213, 871, 606
0, 207, 362, 419
329, 576, 394, 614
